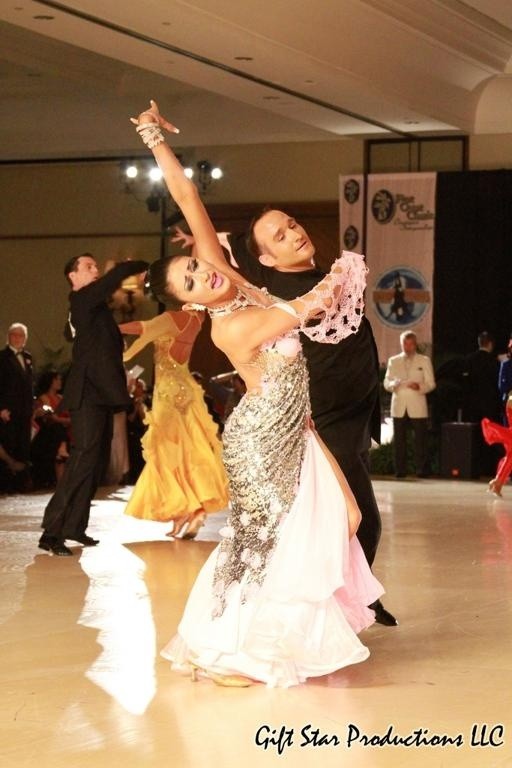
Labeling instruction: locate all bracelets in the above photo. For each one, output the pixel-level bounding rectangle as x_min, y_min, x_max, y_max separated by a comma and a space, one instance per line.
136, 111, 166, 149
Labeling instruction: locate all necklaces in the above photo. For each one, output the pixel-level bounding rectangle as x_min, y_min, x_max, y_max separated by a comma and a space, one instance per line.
207, 283, 258, 320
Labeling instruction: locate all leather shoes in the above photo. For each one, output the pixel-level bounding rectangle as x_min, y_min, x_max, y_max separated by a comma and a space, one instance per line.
368, 599, 398, 626
38, 530, 99, 556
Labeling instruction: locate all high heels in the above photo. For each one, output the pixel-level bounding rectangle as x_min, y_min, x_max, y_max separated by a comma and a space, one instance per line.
166, 510, 207, 539
489, 480, 503, 497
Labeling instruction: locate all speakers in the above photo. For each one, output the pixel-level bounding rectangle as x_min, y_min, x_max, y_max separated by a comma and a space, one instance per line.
442, 423, 478, 480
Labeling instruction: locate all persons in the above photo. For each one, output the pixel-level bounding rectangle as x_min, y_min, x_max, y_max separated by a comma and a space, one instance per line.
497, 337, 512, 425
0, 321, 247, 491
224, 197, 400, 630
481, 390, 512, 497
383, 271, 418, 321
117, 224, 232, 541
464, 329, 504, 477
129, 95, 362, 682
36, 251, 149, 557
382, 329, 437, 479
